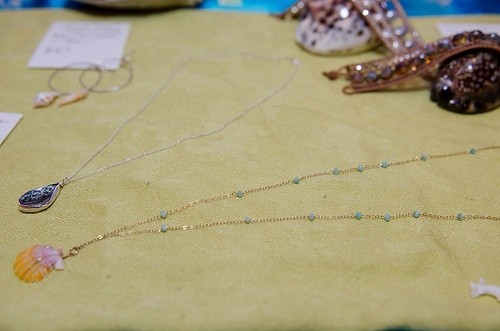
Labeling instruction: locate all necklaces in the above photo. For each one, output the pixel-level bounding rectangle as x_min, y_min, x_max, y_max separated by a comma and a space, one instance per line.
13, 48, 500, 283
